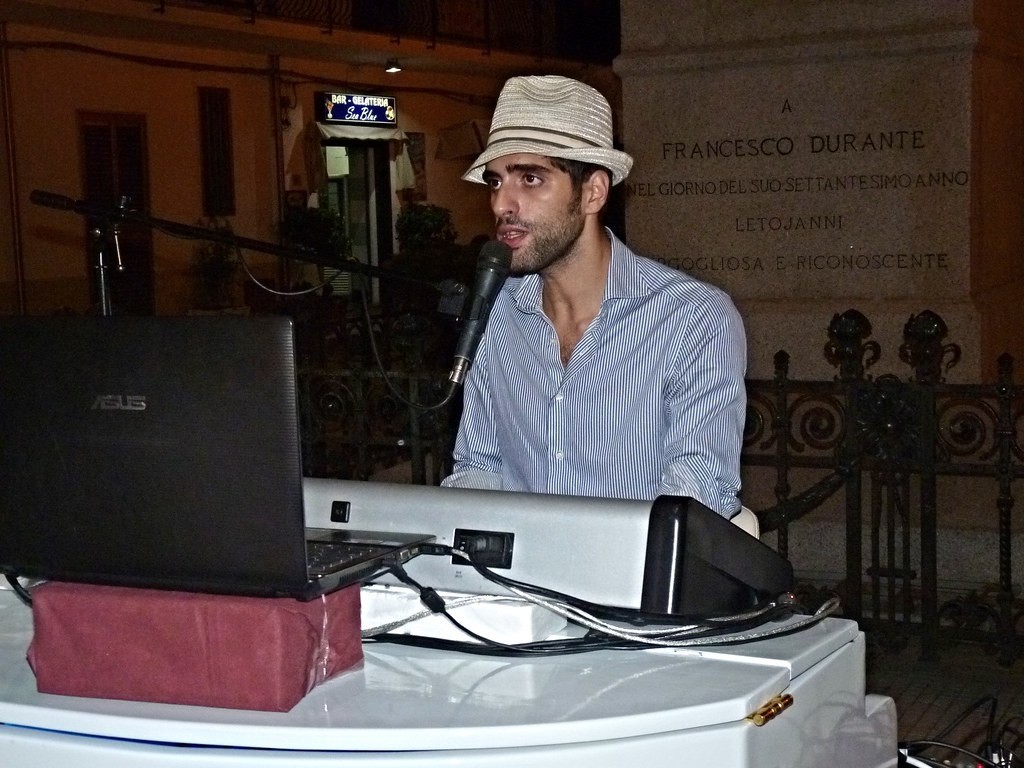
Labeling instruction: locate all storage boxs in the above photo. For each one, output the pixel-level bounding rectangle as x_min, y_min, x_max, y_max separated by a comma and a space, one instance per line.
25, 583, 362, 713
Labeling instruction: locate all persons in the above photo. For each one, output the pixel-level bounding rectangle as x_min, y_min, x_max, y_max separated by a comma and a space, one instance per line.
439, 77, 747, 519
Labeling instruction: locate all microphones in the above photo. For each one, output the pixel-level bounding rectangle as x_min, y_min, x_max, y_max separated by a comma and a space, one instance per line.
445, 240, 513, 399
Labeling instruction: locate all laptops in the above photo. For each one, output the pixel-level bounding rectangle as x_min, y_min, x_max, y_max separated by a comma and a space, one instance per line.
0, 313, 437, 600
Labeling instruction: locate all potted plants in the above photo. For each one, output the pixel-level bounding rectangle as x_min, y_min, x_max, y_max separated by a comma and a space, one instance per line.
277, 206, 352, 296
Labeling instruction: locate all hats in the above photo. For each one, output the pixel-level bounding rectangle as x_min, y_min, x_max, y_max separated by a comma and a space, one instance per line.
461, 74, 634, 189
